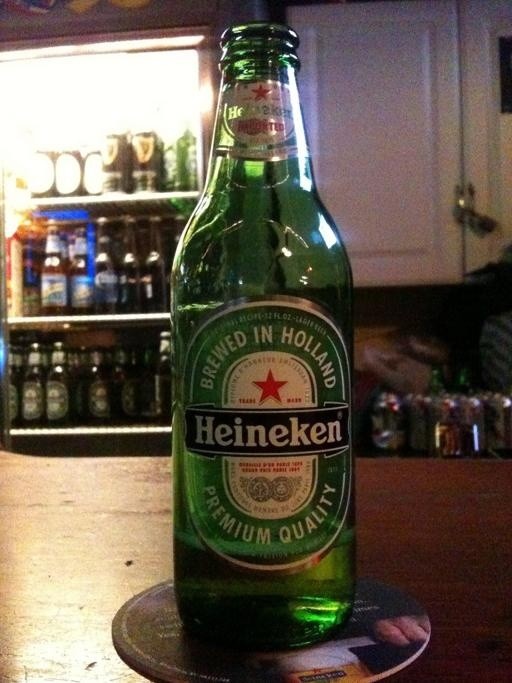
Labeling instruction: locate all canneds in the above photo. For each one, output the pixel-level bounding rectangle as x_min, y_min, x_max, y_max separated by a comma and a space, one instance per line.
30, 132, 196, 198
370, 392, 512, 459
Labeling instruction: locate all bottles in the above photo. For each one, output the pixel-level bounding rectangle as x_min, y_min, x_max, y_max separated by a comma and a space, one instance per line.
10, 330, 175, 423
165, 20, 360, 648
15, 214, 165, 313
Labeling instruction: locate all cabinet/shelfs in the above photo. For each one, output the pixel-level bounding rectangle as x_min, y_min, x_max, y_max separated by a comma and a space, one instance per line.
277, 0, 510, 285
1, 190, 199, 454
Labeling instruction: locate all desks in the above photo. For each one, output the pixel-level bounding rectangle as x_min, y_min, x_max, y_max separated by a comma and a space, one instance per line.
0, 451, 512, 683
